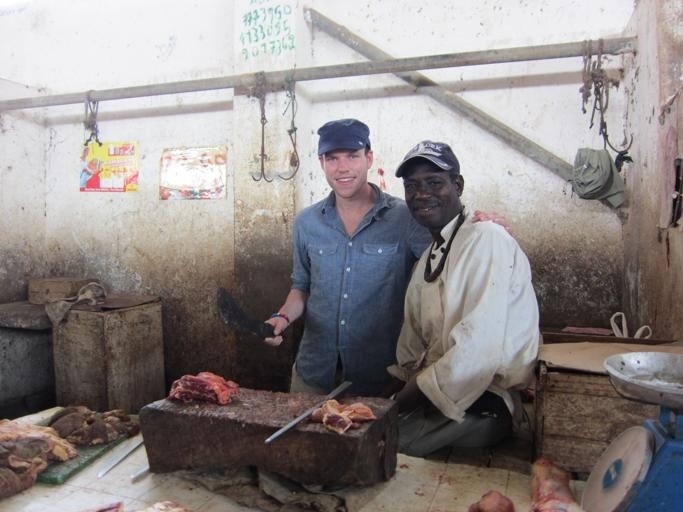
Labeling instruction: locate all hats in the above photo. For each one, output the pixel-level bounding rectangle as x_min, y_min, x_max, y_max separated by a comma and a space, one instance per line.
396, 141, 459, 178
317, 119, 370, 156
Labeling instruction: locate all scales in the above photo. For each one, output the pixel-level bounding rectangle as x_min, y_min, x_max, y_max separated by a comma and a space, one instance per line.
581, 352, 683, 512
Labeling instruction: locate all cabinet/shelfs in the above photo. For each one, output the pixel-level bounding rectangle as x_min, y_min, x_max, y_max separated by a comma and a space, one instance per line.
533, 335, 683, 476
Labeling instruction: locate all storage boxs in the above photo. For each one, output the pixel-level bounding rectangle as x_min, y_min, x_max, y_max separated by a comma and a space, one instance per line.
27, 275, 99, 305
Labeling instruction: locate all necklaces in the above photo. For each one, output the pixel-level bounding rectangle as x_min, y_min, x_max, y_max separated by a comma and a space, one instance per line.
423, 207, 466, 282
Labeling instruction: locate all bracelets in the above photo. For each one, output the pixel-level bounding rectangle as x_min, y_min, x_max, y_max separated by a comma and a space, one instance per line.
269, 313, 290, 324
388, 393, 396, 401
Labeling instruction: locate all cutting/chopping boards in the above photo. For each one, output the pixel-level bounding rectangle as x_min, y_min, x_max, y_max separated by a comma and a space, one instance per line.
28, 404, 140, 486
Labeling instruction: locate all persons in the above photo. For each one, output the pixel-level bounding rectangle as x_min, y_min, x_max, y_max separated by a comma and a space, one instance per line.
257, 117, 514, 401
377, 139, 540, 467
